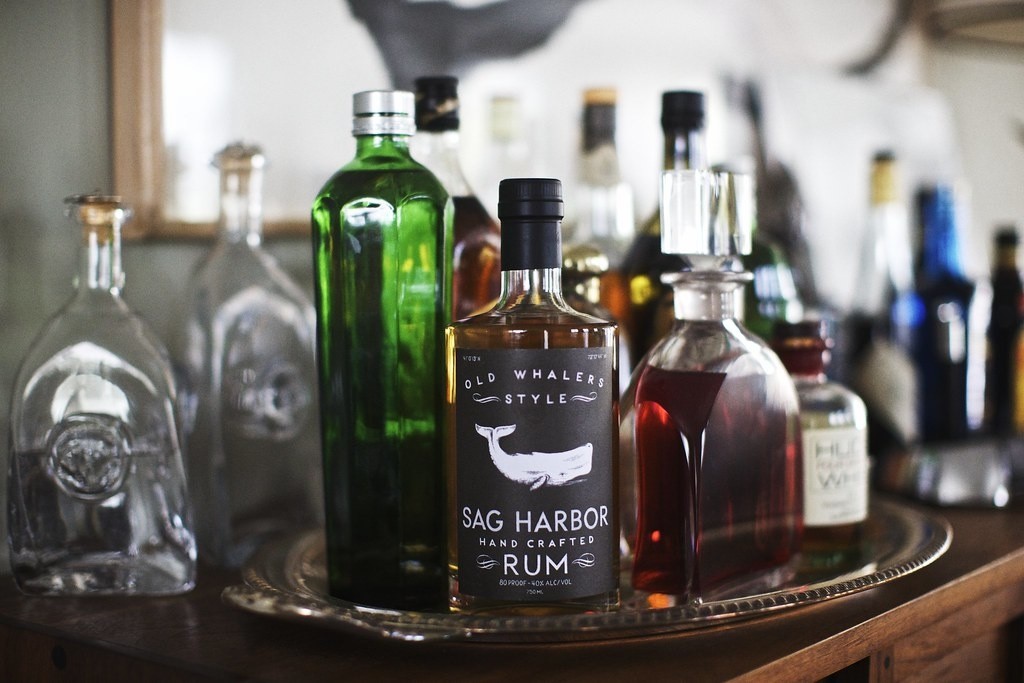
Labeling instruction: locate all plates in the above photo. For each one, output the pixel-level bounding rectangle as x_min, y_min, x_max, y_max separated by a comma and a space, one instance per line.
226, 502, 953, 642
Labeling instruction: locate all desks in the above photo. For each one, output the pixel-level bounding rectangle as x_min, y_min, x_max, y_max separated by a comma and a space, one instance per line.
0, 432, 1024, 683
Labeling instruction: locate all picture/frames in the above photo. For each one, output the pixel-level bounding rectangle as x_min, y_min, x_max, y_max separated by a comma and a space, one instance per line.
107, 0, 315, 248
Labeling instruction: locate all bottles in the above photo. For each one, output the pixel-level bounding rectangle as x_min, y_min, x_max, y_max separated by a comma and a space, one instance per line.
7, 73, 1024, 617
